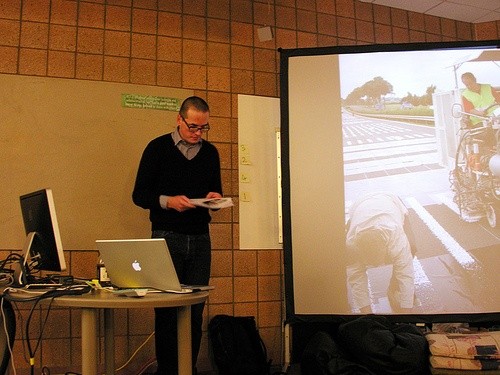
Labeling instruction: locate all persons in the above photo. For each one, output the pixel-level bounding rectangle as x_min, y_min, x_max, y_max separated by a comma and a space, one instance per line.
132, 96, 223, 375
344, 192, 418, 314
461, 72, 500, 139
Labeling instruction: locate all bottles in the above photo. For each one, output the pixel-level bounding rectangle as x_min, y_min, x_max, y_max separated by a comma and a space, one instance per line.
97, 255, 112, 287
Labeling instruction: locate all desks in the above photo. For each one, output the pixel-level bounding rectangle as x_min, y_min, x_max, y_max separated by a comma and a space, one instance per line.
1, 279, 209, 375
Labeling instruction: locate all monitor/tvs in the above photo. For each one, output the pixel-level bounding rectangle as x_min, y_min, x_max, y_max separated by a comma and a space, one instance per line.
13, 187, 66, 285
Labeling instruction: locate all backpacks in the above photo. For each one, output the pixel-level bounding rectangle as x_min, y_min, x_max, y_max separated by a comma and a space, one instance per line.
210, 315, 270, 375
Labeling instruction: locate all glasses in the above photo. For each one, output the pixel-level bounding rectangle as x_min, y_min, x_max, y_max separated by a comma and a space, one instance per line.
180, 114, 210, 132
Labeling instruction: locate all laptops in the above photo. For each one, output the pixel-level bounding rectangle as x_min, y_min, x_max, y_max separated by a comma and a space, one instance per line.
96, 238, 215, 292
